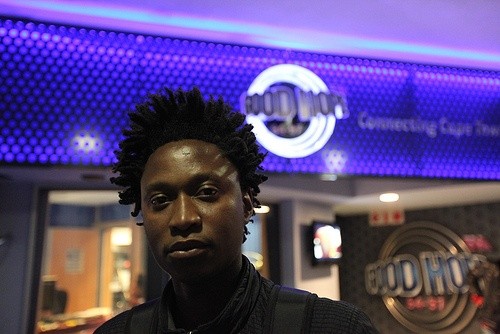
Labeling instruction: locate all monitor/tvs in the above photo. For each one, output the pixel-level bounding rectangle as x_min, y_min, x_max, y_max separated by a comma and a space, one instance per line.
311, 220, 343, 265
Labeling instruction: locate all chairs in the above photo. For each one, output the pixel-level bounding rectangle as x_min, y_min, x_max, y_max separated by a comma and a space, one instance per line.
56, 290, 67, 314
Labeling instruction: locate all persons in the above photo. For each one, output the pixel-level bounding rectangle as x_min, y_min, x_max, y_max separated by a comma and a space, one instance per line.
93, 86, 380, 333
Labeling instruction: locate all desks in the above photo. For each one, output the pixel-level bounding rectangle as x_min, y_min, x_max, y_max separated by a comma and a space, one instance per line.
35, 315, 114, 334
